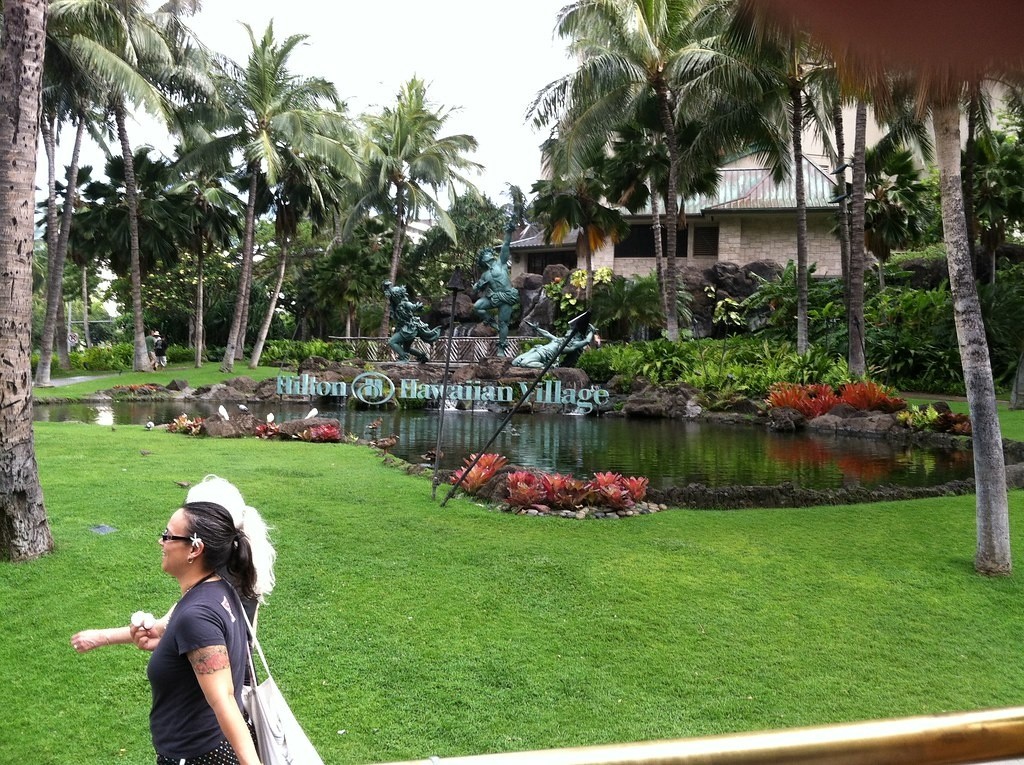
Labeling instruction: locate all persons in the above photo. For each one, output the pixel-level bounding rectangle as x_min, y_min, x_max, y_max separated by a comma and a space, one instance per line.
470, 214, 520, 359
511, 316, 598, 367
69, 474, 276, 687
128, 502, 263, 765
382, 280, 442, 364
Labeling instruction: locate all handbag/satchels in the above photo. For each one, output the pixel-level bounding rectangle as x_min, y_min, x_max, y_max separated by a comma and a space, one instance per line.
243, 677, 326, 765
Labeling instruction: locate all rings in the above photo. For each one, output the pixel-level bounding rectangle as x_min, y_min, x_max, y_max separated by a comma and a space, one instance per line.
73, 645, 78, 650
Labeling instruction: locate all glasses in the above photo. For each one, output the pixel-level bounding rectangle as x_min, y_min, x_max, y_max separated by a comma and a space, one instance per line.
162, 530, 192, 543
